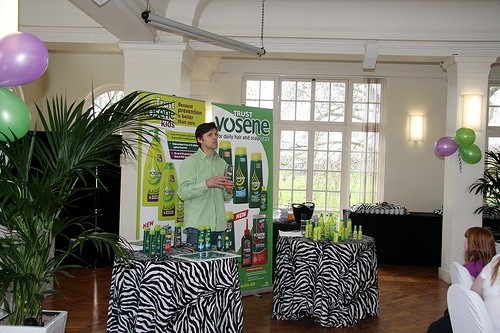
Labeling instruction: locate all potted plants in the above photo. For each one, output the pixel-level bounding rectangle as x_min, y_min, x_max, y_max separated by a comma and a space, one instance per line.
0, 76, 178, 333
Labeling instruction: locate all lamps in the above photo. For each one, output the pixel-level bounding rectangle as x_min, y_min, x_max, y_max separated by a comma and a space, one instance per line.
408, 112, 425, 142
141, 0, 266, 58
463, 94, 483, 132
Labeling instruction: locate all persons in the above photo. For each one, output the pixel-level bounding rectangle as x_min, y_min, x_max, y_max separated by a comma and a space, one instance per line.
177, 122, 234, 251
426, 226, 500, 333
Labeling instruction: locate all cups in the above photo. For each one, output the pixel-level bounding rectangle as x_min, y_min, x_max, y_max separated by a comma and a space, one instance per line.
287, 211, 294, 221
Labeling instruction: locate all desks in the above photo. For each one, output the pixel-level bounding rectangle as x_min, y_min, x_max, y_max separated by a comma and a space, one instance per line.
272, 221, 314, 285
271, 233, 380, 329
342, 208, 444, 270
106, 243, 244, 333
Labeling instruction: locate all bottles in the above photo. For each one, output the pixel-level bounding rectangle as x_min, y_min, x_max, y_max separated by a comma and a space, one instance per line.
300, 213, 362, 243
217, 235, 223, 251
349, 201, 410, 215
174, 222, 183, 249
204, 225, 211, 250
219, 139, 268, 212
241, 214, 266, 267
143, 128, 184, 222
224, 236, 230, 251
143, 224, 171, 261
197, 226, 205, 252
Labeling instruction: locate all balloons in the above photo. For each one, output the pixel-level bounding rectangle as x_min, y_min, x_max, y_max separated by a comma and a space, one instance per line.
0, 32, 49, 141
434, 126, 482, 165
